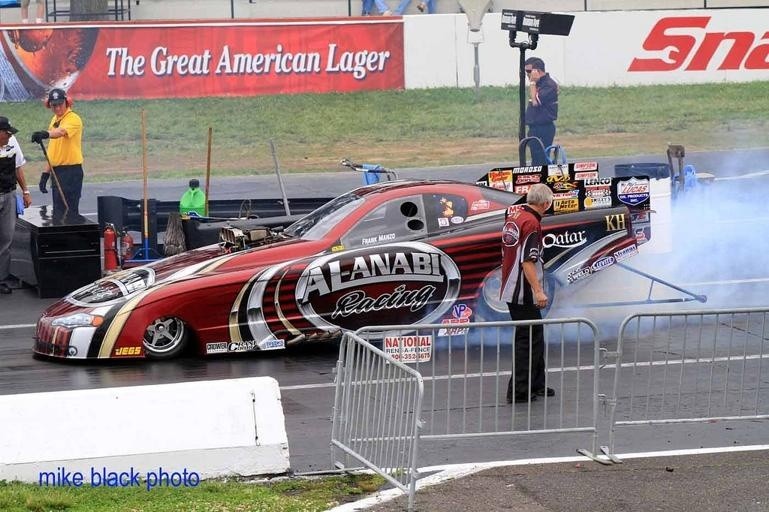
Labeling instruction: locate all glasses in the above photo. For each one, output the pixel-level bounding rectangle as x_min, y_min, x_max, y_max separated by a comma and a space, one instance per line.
524, 69, 533, 74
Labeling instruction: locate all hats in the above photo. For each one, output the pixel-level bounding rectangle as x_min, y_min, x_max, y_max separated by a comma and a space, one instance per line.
0, 116, 19, 134
49, 89, 66, 106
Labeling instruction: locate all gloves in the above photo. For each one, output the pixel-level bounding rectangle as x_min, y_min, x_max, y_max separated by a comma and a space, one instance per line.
31, 131, 49, 142
40, 173, 50, 194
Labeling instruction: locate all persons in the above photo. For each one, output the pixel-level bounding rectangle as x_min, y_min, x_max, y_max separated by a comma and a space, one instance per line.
494, 181, 555, 405
522, 57, 560, 165
0, 114, 33, 298
19, 0, 46, 25
33, 87, 85, 217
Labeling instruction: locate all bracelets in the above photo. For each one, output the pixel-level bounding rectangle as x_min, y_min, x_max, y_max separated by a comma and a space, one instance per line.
22, 189, 31, 196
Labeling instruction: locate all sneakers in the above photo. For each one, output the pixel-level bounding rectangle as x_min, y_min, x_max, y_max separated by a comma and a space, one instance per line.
0, 284, 12, 294
507, 393, 536, 402
537, 387, 554, 396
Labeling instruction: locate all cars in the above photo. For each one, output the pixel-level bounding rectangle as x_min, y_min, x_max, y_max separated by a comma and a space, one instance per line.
32, 180, 638, 359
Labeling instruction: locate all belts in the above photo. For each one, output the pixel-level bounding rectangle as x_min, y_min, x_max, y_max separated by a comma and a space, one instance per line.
0, 186, 17, 193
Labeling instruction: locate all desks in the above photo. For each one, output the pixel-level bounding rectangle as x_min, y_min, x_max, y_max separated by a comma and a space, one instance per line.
8, 203, 102, 299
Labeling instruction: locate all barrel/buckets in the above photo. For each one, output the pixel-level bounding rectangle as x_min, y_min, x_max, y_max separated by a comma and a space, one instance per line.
178, 178, 209, 216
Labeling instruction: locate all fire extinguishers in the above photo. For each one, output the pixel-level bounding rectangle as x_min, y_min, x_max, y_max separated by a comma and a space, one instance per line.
120, 226, 133, 265
104, 221, 117, 271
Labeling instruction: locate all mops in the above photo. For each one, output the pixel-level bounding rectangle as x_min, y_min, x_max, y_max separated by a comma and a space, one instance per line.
163, 210, 247, 257
121, 107, 162, 268
